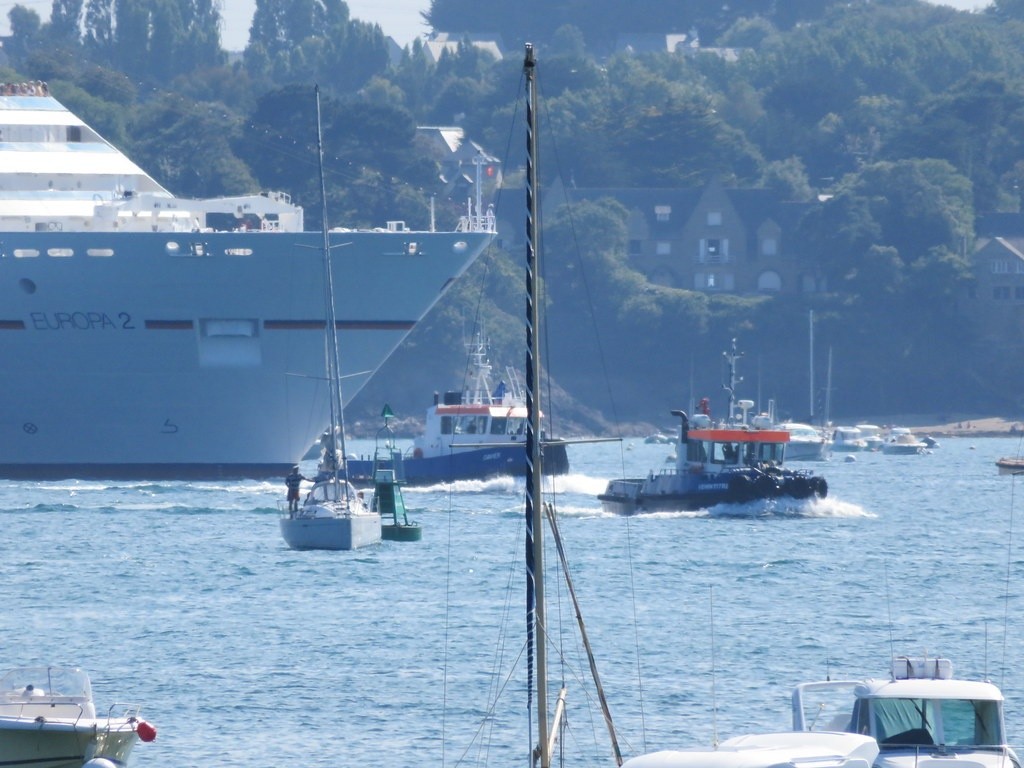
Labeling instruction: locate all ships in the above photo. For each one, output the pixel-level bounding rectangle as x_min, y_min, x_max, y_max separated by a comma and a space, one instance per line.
0, 81, 499, 480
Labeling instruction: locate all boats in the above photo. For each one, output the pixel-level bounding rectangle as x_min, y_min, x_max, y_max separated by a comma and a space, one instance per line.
596, 335, 829, 504
790, 654, 1021, 766
775, 420, 929, 456
995, 455, 1024, 477
307, 311, 571, 485
0, 664, 156, 767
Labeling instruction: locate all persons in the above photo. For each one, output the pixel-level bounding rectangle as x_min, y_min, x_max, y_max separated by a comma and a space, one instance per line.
285, 467, 311, 519
0, 80, 49, 97
517, 423, 523, 434
467, 421, 476, 433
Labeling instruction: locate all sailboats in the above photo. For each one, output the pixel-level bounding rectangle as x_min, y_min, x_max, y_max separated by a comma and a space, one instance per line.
515, 38, 878, 766
277, 84, 423, 545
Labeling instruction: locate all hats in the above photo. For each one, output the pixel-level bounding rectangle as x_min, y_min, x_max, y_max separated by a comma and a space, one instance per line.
292, 466, 299, 469
